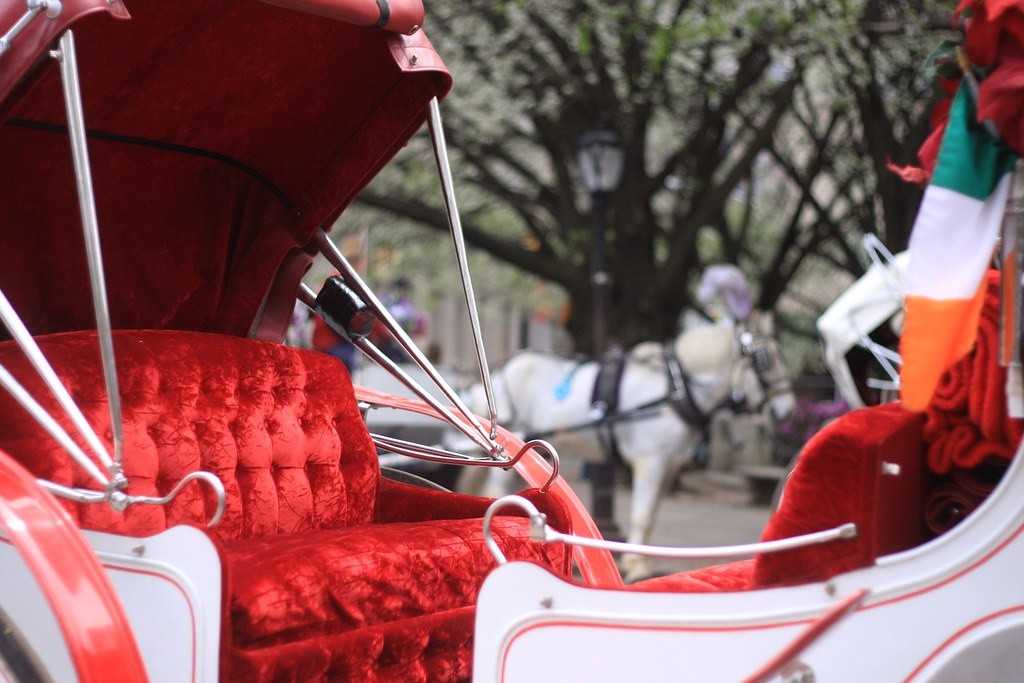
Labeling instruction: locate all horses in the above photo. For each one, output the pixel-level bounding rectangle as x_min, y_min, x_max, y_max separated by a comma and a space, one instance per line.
457, 308, 796, 583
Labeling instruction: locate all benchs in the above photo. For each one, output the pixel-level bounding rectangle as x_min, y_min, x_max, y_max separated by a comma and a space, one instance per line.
472, 403, 930, 683
0, 329, 573, 683
